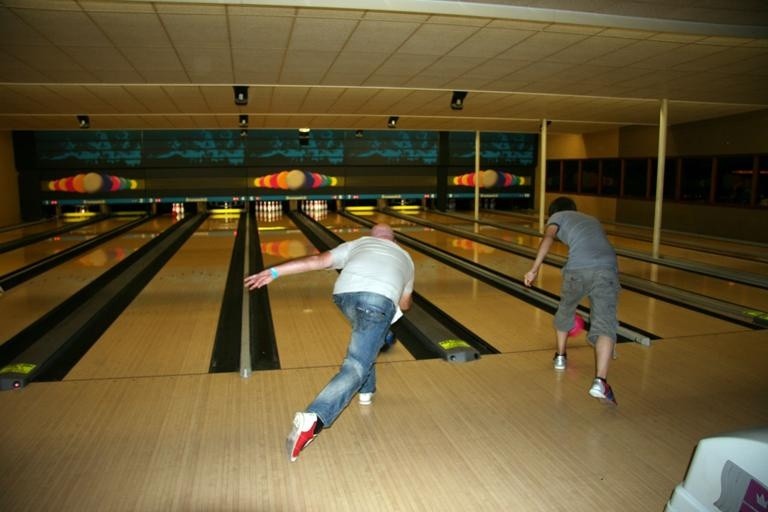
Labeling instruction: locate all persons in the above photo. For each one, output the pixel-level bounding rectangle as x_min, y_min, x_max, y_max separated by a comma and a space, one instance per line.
524, 196, 620, 404
242, 222, 414, 463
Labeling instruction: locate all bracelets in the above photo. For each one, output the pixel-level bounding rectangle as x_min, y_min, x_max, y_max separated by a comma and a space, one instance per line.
269, 267, 279, 281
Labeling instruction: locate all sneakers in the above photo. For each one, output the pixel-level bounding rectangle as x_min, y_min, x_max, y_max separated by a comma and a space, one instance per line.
552, 356, 566, 370
287, 412, 317, 462
360, 393, 374, 405
588, 378, 618, 407
